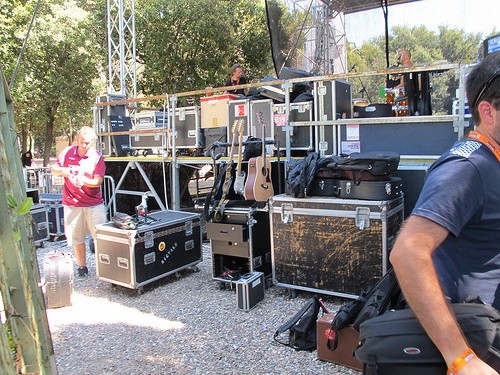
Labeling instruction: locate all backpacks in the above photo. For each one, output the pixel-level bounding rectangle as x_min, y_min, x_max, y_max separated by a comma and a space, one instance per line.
274, 295, 330, 351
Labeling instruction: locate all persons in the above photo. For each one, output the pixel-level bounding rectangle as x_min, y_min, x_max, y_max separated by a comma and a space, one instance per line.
46, 126, 110, 277
393, 49, 414, 89
388, 51, 500, 375
226, 66, 249, 95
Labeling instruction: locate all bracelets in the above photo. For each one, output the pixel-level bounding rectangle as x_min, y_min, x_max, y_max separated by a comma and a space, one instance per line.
443, 349, 478, 375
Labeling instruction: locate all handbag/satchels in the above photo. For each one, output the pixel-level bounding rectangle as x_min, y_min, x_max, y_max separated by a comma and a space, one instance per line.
327, 268, 402, 351
354, 302, 500, 375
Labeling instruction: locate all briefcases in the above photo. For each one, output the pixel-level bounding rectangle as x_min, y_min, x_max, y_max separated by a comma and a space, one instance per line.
236, 271, 265, 311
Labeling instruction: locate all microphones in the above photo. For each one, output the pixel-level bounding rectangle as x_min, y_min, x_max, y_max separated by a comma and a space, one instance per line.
349, 64, 356, 72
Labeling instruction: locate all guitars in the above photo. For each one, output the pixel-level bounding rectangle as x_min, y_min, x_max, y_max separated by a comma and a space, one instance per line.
243, 110, 275, 201
213, 119, 239, 199
226, 119, 247, 201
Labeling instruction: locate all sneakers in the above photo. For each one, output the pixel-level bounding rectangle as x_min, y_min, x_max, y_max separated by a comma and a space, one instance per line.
77, 266, 88, 277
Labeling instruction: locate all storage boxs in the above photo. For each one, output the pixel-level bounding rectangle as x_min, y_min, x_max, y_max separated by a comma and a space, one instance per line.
236, 272, 265, 311
26, 189, 64, 244
208, 207, 272, 283
168, 105, 204, 148
314, 79, 352, 158
269, 191, 404, 299
273, 101, 313, 150
250, 99, 273, 156
226, 98, 250, 156
93, 210, 202, 289
98, 94, 126, 157
129, 112, 167, 149
200, 93, 237, 128
